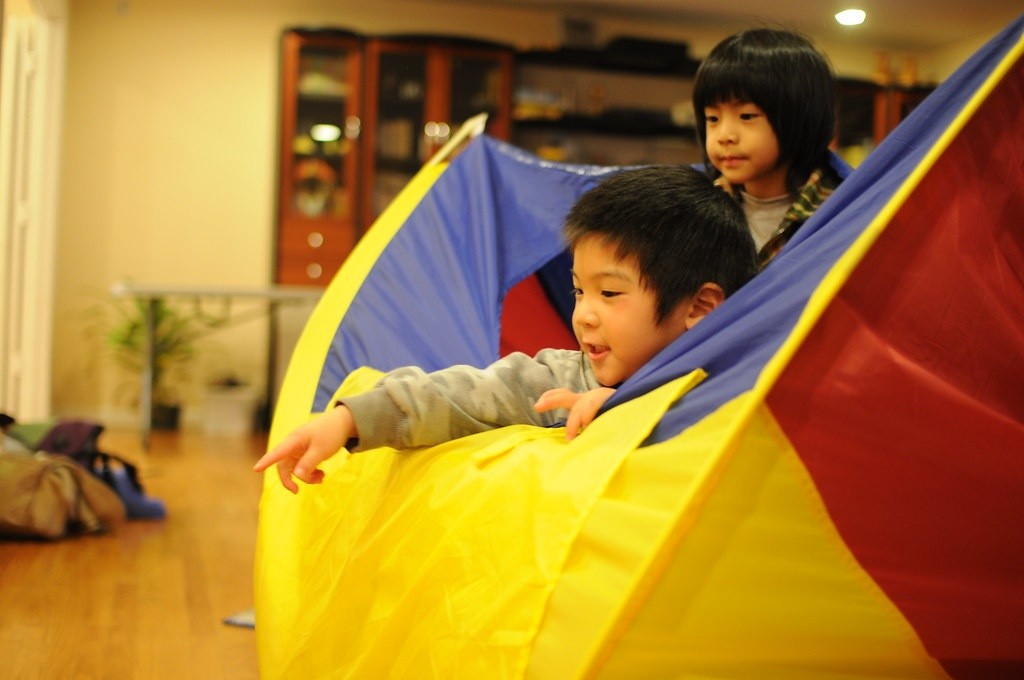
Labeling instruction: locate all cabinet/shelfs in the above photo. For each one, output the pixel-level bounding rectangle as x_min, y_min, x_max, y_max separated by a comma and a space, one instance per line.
268, 25, 938, 423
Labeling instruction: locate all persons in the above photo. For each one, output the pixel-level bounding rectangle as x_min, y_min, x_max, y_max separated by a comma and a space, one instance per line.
691, 29, 845, 268
253, 162, 758, 494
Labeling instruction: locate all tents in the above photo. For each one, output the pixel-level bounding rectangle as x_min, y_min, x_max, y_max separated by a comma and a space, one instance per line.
255, 19, 1024, 680
